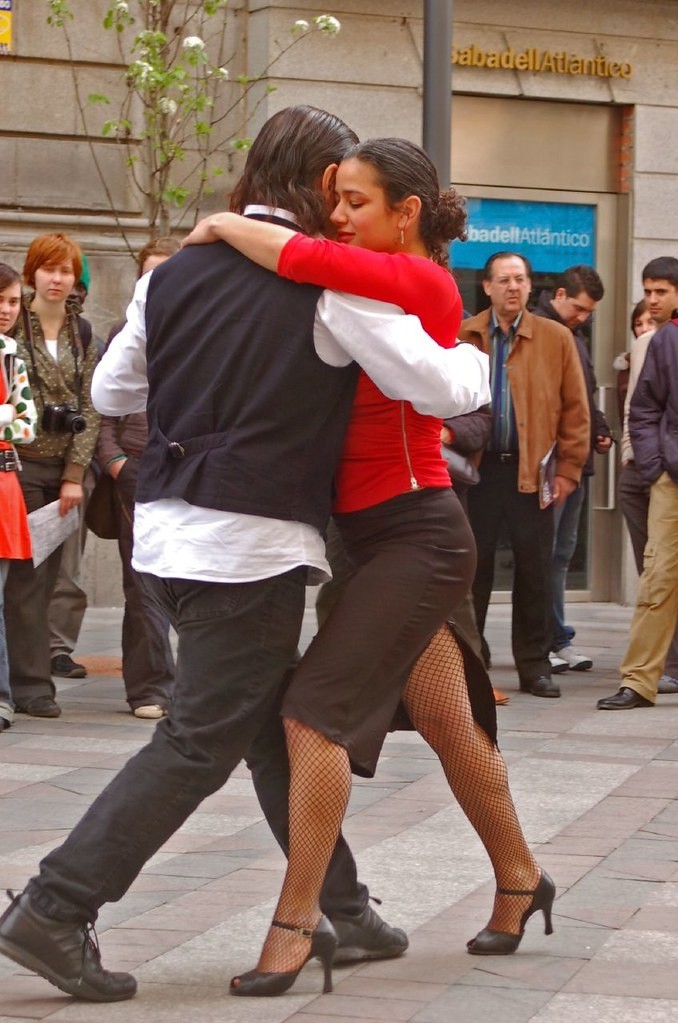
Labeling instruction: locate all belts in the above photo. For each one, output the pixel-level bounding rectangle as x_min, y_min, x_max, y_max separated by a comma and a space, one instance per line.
0, 448, 19, 471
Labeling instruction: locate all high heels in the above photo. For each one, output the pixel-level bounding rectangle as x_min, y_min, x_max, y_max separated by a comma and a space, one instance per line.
467, 866, 557, 954
230, 914, 337, 997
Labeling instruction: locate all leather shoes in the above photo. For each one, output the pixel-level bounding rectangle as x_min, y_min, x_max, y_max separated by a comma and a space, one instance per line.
13, 697, 60, 716
596, 687, 654, 709
519, 675, 561, 697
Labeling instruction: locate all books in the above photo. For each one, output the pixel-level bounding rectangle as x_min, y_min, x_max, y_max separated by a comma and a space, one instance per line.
539, 440, 554, 510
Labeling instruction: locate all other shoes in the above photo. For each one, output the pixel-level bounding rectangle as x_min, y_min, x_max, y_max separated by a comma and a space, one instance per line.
0, 716, 9, 730
494, 689, 510, 705
134, 704, 162, 718
657, 675, 678, 693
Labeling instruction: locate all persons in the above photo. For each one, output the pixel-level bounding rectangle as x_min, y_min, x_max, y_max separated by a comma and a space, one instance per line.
0, 231, 181, 734
454, 249, 591, 697
597, 252, 678, 711
432, 403, 495, 513
178, 136, 554, 996
0, 103, 494, 1002
526, 264, 615, 673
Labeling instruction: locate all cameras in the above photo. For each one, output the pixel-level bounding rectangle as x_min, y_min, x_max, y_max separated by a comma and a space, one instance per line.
42, 405, 87, 434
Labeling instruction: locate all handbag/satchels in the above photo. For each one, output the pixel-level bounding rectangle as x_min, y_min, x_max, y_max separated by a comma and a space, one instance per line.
82, 472, 119, 540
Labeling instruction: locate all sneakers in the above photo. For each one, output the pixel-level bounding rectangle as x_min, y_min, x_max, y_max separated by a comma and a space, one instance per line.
327, 896, 409, 963
549, 652, 570, 673
0, 888, 139, 1000
51, 654, 87, 677
556, 646, 593, 669
